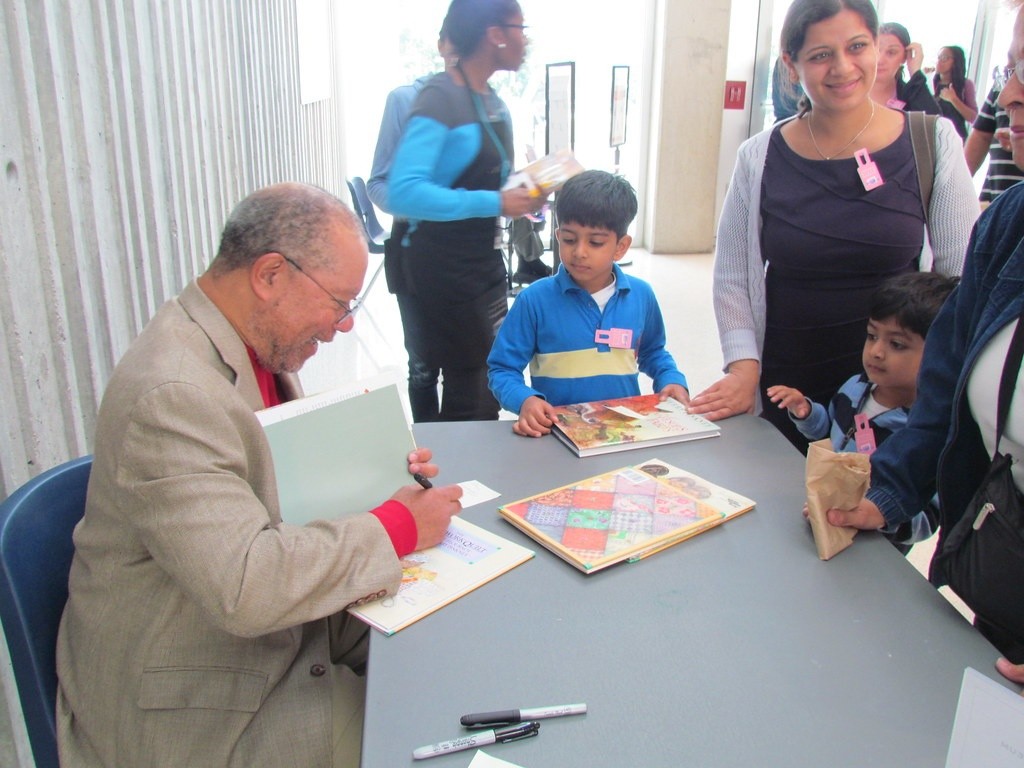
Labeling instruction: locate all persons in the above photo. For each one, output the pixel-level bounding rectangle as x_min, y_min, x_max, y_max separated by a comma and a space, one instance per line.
55, 181, 462, 768
366, 0, 547, 423
686, 0, 1024, 687
486, 169, 692, 437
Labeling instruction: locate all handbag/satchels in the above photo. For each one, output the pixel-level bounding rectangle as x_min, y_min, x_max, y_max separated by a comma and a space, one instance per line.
932, 452, 1024, 644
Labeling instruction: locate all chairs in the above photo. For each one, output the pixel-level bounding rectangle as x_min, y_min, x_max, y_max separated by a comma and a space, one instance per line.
0, 454, 92, 768
343, 174, 393, 312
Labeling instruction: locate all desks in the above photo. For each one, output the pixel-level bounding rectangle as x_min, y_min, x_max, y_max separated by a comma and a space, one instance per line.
361, 397, 1024, 768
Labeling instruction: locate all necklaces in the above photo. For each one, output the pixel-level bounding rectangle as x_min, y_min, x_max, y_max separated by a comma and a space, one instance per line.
808, 99, 874, 161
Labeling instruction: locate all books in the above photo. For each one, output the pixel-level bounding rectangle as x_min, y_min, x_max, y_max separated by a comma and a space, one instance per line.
254, 374, 534, 636
504, 149, 584, 196
546, 394, 721, 458
499, 456, 757, 574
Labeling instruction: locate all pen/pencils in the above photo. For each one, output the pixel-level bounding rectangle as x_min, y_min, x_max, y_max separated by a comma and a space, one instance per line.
460, 702, 590, 728
413, 473, 434, 489
411, 722, 539, 761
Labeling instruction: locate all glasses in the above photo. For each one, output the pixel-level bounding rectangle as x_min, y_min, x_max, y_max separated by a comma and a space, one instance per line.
255, 249, 360, 324
936, 54, 959, 63
485, 23, 531, 39
993, 60, 1024, 90
